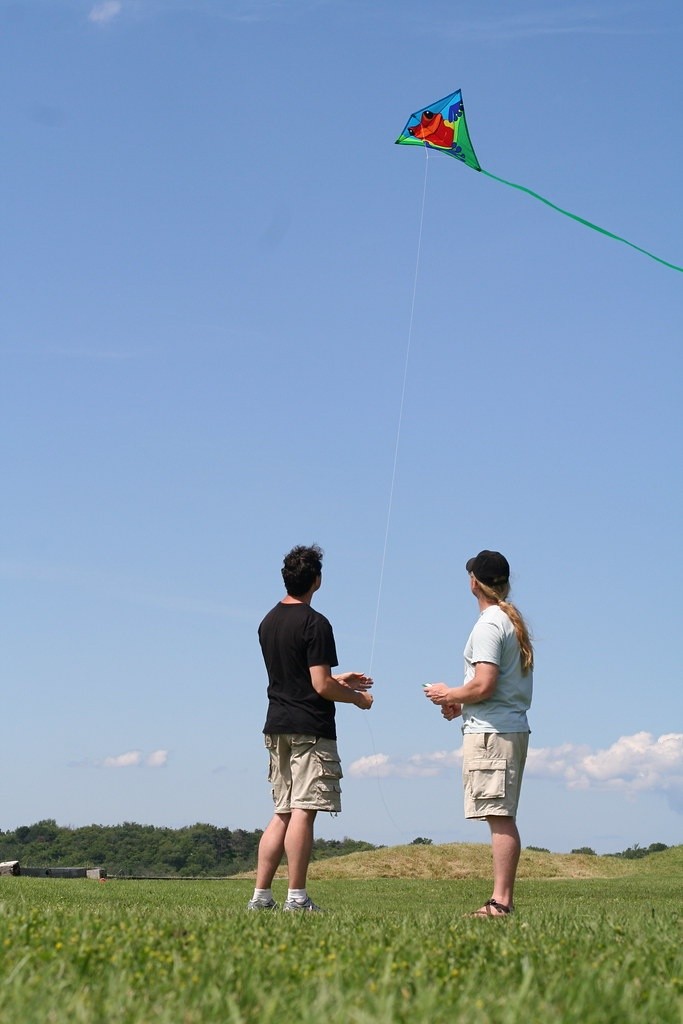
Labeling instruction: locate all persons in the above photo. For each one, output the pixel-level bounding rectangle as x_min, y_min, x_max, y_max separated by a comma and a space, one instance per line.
424, 548, 532, 916
240, 546, 372, 913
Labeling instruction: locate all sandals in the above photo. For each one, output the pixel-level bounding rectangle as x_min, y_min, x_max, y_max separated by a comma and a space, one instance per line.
469, 899, 511, 919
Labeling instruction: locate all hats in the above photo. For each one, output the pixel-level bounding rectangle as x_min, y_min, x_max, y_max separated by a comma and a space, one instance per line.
466, 549, 511, 588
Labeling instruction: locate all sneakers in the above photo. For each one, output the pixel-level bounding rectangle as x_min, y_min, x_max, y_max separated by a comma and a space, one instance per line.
283, 895, 324, 914
247, 899, 280, 910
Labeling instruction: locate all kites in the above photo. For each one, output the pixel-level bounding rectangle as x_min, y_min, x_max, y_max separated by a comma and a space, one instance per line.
394, 88, 484, 173
